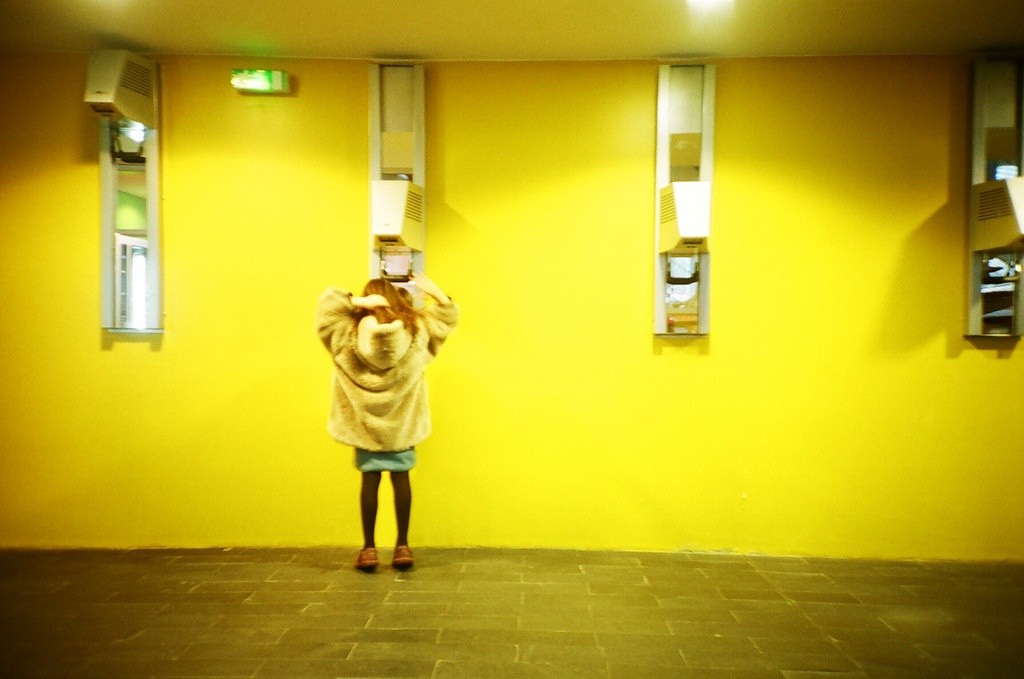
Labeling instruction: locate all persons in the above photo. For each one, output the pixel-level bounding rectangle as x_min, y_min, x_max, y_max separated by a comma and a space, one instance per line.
315, 269, 458, 569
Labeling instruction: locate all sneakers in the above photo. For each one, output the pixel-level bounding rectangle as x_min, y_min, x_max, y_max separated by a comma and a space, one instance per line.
393, 546, 414, 565
355, 549, 380, 566
